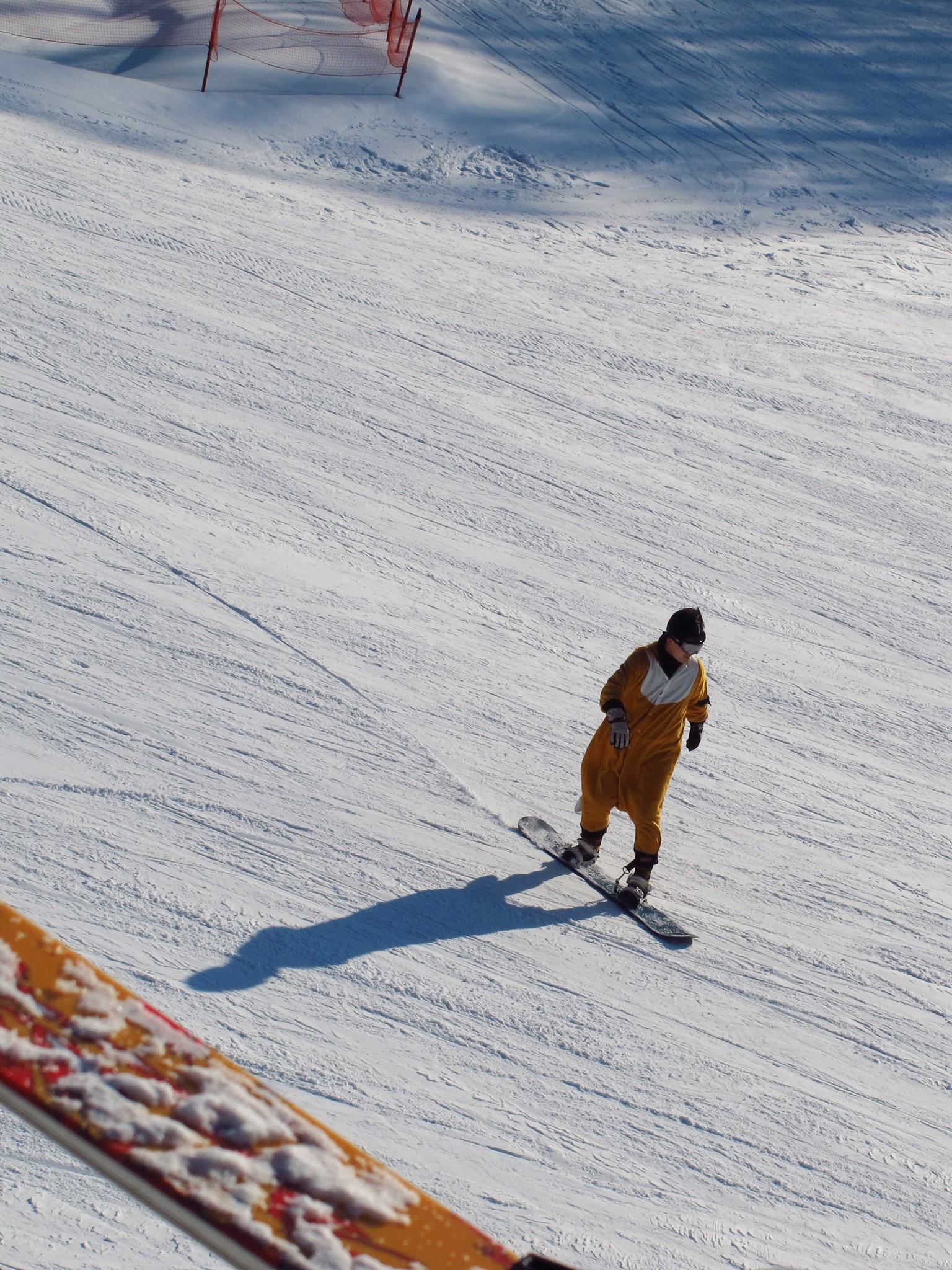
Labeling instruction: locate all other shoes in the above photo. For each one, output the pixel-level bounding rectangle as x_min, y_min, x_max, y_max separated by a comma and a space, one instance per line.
618, 869, 649, 904
562, 835, 602, 862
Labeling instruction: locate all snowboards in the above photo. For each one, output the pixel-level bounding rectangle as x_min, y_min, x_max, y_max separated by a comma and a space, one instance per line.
0, 902, 576, 1270
518, 816, 700, 938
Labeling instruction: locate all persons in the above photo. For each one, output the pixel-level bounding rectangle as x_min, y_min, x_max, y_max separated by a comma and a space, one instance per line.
563, 608, 711, 906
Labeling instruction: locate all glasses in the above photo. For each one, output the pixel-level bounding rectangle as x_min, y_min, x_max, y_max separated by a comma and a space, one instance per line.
680, 641, 703, 654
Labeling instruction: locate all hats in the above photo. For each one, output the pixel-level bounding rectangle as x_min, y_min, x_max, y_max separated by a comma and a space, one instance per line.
667, 608, 703, 642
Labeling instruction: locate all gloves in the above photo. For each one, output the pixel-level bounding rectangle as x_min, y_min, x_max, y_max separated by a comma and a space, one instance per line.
685, 720, 706, 751
606, 707, 630, 750
